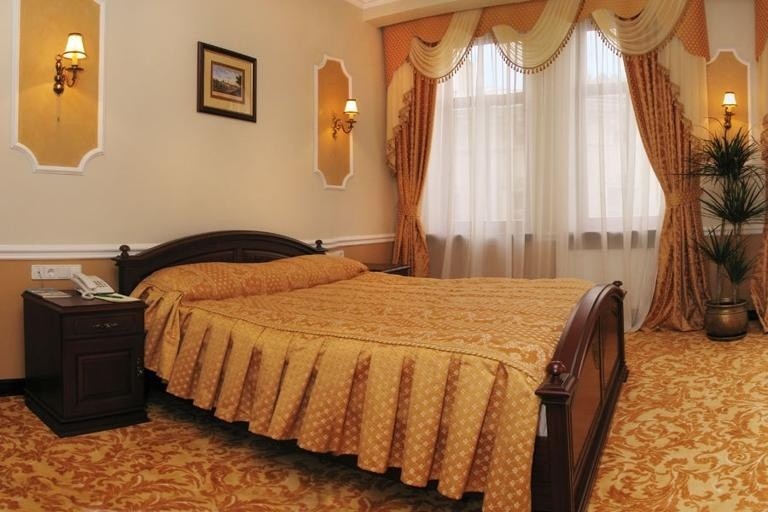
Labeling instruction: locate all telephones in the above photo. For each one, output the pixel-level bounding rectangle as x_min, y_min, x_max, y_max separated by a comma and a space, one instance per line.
71, 273, 114, 295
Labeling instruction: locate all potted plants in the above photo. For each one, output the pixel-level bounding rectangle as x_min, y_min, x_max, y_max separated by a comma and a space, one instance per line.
662, 115, 768, 342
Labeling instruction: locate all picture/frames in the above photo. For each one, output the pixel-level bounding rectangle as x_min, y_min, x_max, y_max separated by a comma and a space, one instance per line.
197, 41, 256, 121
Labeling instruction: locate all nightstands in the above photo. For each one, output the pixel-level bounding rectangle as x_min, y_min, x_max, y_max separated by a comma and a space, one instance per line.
20, 290, 148, 438
361, 262, 411, 276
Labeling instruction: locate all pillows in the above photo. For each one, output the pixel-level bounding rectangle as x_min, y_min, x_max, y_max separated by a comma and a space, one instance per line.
128, 253, 368, 382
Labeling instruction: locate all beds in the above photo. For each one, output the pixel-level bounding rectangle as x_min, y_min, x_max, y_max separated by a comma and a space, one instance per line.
111, 229, 629, 512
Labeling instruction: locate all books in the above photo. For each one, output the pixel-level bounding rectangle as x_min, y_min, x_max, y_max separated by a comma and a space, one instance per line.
30, 287, 71, 299
95, 293, 140, 303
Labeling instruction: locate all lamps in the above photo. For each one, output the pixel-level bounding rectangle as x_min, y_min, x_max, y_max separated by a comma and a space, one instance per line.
722, 91, 738, 129
54, 33, 90, 94
331, 99, 360, 139
